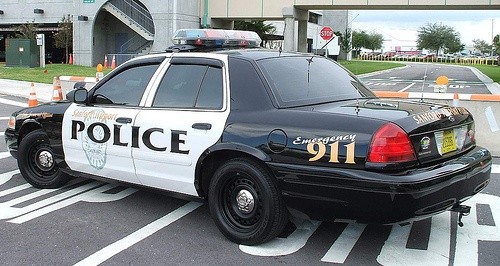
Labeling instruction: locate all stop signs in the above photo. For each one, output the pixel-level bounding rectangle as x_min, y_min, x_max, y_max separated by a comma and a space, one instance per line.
319, 26, 333, 40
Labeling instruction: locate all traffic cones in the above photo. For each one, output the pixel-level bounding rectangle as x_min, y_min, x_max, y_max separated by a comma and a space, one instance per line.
51, 77, 60, 101
28, 82, 38, 106
112, 56, 116, 68
69, 53, 72, 64
104, 55, 108, 68
56, 75, 63, 100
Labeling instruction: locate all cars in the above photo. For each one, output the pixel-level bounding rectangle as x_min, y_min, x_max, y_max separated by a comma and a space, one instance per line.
3, 28, 492, 245
